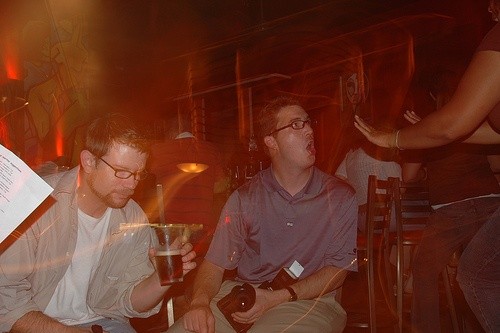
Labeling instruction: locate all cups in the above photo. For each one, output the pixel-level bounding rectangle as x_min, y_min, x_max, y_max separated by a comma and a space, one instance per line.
149, 226, 184, 286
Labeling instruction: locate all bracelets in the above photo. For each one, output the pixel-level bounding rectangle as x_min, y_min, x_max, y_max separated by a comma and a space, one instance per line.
396, 128, 403, 153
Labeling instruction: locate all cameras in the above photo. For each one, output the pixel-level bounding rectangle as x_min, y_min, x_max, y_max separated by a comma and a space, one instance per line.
231, 282, 256, 314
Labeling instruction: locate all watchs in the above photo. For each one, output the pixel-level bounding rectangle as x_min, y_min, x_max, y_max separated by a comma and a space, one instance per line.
287, 287, 297, 302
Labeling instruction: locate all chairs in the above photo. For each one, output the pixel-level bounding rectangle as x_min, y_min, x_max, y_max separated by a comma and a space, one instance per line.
342, 175, 459, 333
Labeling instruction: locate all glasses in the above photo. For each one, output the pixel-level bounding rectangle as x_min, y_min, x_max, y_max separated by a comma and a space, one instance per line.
98, 155, 149, 181
271, 114, 314, 135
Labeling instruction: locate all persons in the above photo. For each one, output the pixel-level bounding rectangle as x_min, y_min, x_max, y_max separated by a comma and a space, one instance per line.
166, 96, 358, 333
353, 0, 500, 332
0, 113, 197, 333
335, 90, 432, 231
